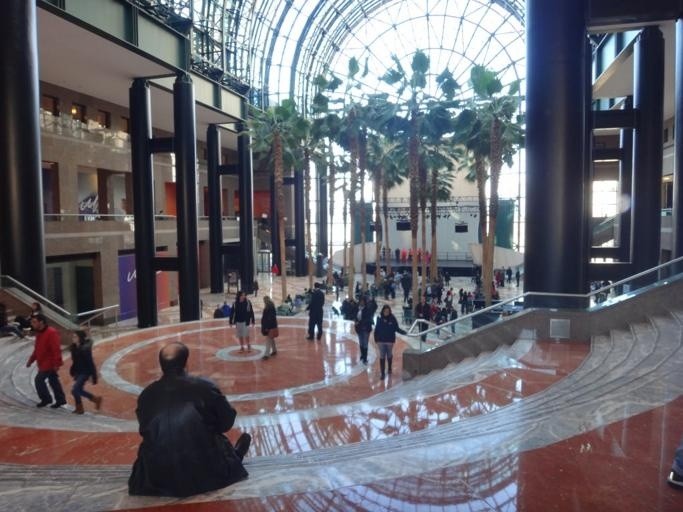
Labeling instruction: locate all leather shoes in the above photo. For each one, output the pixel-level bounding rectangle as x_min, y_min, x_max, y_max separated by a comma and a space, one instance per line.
235, 432, 251, 463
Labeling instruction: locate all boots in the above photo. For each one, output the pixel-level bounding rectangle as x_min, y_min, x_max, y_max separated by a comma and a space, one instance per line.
90, 395, 102, 411
359, 346, 368, 365
72, 404, 84, 414
379, 358, 386, 380
387, 356, 393, 374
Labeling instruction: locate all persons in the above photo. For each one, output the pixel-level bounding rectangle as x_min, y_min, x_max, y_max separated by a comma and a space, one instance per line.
213, 304, 224, 318
221, 301, 231, 317
230, 290, 254, 354
0, 303, 27, 338
666, 440, 682, 489
274, 245, 520, 340
259, 296, 277, 360
14, 301, 41, 331
590, 277, 612, 306
372, 304, 407, 381
123, 342, 252, 499
68, 331, 102, 414
352, 296, 374, 363
304, 282, 324, 340
23, 313, 66, 408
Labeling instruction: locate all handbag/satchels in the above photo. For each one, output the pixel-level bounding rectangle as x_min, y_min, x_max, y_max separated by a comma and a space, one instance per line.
268, 328, 279, 338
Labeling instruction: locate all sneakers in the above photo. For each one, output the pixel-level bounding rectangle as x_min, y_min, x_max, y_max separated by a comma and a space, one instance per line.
306, 335, 314, 339
36, 396, 53, 408
50, 395, 67, 408
261, 356, 269, 361
316, 333, 321, 340
247, 344, 251, 352
271, 352, 276, 356
238, 348, 244, 353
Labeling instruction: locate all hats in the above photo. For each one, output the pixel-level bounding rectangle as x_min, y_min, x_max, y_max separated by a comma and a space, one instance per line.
74, 331, 86, 343
30, 312, 46, 321
